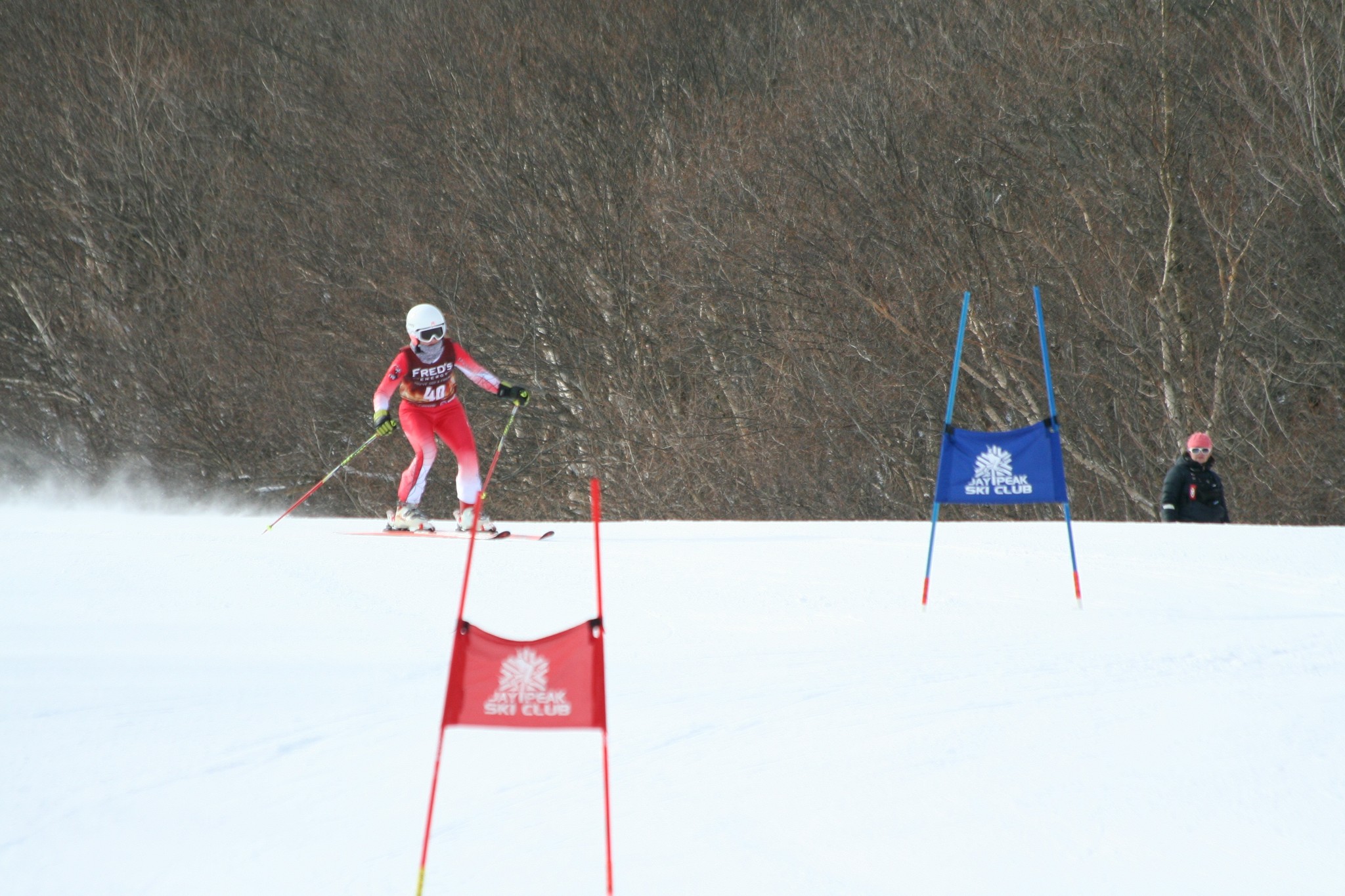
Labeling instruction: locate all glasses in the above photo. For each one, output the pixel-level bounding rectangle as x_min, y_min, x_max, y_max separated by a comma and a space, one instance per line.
1189, 447, 1211, 454
419, 325, 445, 342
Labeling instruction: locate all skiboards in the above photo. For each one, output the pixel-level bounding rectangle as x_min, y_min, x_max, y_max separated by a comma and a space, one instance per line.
336, 530, 555, 540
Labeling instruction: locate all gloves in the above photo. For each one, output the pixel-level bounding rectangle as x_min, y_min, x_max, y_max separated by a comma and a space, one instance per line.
374, 410, 396, 437
497, 381, 529, 407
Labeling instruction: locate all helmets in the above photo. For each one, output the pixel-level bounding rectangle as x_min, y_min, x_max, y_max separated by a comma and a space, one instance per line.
406, 304, 445, 337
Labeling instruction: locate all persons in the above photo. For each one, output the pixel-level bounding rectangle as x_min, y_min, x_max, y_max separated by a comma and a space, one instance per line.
374, 305, 530, 536
1159, 433, 1231, 523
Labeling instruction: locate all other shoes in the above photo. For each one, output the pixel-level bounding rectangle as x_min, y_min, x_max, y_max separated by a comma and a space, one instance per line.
395, 507, 423, 530
461, 507, 492, 531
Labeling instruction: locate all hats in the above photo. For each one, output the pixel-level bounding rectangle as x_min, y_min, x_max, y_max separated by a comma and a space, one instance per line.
1187, 432, 1212, 448
